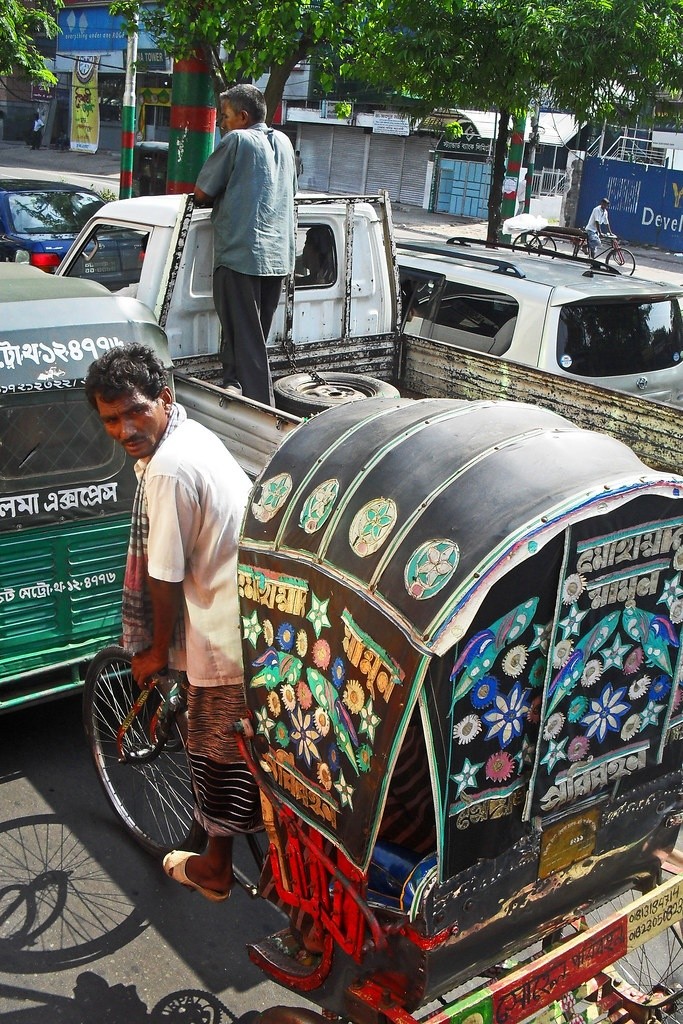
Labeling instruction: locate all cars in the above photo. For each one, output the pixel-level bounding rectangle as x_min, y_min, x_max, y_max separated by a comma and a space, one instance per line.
0, 176, 144, 291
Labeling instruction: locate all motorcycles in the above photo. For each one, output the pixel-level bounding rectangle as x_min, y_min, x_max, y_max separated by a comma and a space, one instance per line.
0, 263, 175, 712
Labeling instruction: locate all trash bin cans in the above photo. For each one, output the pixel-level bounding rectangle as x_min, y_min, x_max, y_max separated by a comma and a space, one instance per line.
132, 140, 169, 199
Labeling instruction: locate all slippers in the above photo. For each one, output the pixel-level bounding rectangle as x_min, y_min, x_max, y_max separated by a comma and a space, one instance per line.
162, 852, 231, 903
580, 247, 588, 255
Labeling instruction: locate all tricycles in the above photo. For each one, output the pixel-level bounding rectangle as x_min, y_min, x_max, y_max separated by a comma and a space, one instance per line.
81, 397, 683, 1023
513, 228, 636, 277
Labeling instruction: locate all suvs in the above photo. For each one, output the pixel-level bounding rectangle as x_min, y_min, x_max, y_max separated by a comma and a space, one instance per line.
394, 236, 683, 410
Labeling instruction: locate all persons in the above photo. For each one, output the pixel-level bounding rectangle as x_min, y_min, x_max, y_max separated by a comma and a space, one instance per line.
581, 198, 613, 258
31, 113, 45, 149
296, 227, 332, 286
84, 344, 257, 900
193, 83, 300, 408
295, 150, 304, 180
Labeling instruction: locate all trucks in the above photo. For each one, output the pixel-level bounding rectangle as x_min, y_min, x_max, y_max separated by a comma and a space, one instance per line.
14, 187, 683, 512
